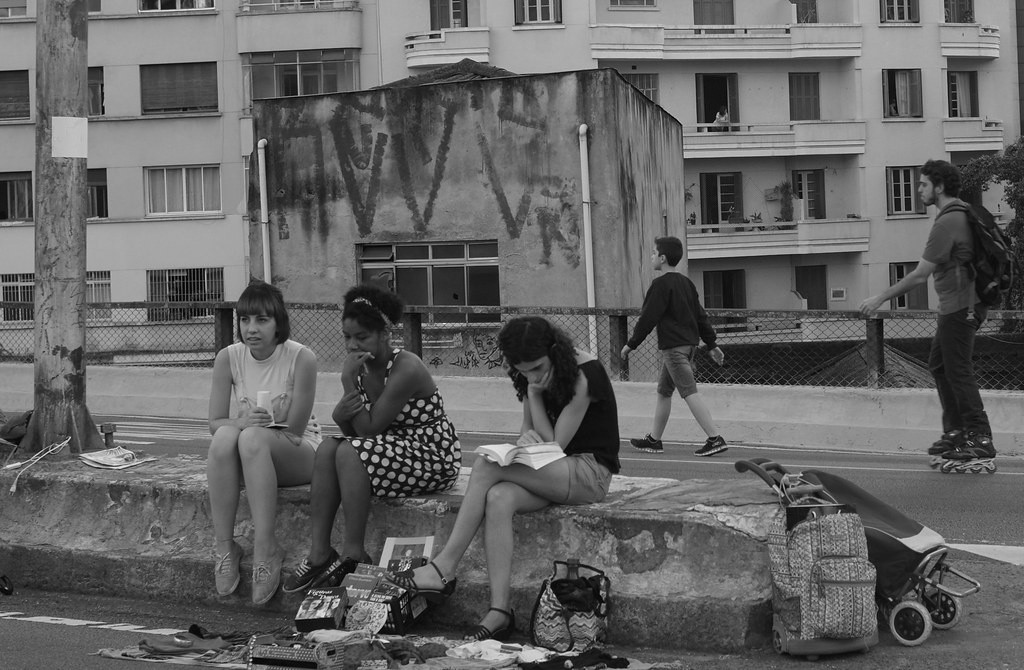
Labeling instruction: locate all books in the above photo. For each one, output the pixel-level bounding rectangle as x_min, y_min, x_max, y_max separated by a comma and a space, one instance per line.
475, 442, 567, 470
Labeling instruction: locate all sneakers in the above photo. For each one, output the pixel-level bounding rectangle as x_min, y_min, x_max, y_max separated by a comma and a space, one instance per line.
282, 547, 340, 593
214, 540, 243, 597
310, 554, 372, 591
251, 544, 285, 605
630, 433, 663, 453
694, 436, 728, 457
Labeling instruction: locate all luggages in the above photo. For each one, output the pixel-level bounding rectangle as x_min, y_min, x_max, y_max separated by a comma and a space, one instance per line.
734, 456, 982, 646
768, 496, 880, 657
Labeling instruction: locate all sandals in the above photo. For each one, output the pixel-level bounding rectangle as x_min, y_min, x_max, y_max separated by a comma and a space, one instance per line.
463, 606, 514, 642
386, 562, 458, 598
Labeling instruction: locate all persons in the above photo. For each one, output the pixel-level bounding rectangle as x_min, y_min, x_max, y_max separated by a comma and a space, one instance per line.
282, 282, 461, 592
206, 280, 322, 603
712, 107, 729, 132
621, 235, 729, 457
859, 160, 998, 474
384, 315, 621, 640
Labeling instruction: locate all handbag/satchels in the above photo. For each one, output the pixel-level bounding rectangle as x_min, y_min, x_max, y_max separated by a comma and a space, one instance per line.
530, 559, 611, 656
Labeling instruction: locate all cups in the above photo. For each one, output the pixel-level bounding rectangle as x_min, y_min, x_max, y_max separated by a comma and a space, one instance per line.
257, 391, 274, 425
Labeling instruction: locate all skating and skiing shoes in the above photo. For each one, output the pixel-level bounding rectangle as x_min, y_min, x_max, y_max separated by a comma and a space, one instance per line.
940, 429, 997, 474
927, 429, 968, 471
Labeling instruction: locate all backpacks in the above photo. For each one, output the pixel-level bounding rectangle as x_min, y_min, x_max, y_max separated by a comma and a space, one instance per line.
937, 202, 1024, 308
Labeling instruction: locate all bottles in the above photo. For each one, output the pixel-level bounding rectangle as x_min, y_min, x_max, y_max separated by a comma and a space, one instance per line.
566, 558, 580, 580
